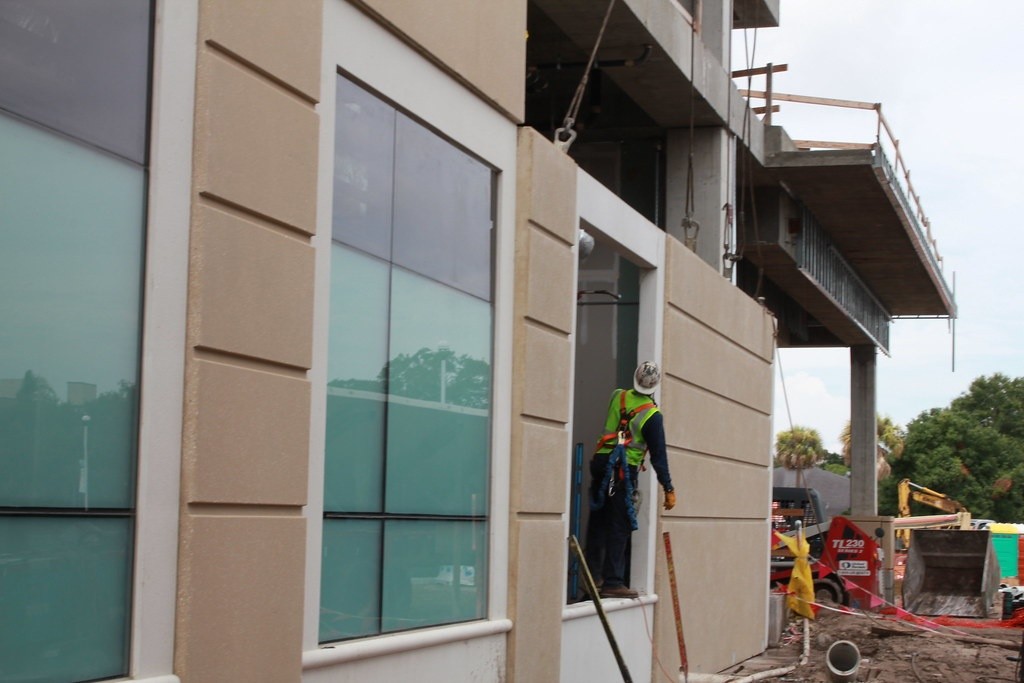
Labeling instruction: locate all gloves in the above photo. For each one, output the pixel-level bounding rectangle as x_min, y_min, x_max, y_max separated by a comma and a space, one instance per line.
664, 491, 676, 511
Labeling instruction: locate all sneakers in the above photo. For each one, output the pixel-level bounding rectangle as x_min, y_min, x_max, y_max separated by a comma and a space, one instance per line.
578, 576, 601, 598
600, 585, 638, 598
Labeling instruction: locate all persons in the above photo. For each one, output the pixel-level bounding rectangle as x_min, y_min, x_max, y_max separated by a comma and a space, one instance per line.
584, 360, 676, 600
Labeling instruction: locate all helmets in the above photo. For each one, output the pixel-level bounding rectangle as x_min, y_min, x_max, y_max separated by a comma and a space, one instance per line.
632, 360, 659, 395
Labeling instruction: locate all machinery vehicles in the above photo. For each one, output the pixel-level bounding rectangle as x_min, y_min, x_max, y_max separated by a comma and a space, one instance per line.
773, 484, 881, 609
888, 477, 970, 557
